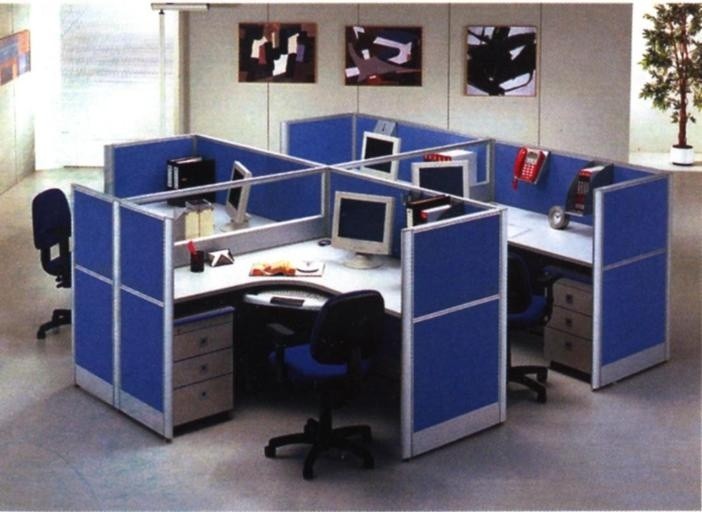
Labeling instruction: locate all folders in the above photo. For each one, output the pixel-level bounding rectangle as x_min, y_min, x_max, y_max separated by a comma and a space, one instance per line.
167, 155, 214, 207
406, 195, 467, 227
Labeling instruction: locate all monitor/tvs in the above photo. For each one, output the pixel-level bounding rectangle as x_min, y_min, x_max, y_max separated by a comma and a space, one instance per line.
218, 160, 252, 234
331, 191, 395, 270
360, 131, 401, 181
411, 159, 470, 198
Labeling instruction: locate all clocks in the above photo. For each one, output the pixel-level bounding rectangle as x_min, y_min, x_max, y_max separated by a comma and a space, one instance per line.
548, 205, 568, 229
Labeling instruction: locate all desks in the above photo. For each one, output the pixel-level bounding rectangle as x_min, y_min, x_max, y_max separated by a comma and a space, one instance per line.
173, 236, 403, 324
141, 199, 275, 238
483, 201, 593, 267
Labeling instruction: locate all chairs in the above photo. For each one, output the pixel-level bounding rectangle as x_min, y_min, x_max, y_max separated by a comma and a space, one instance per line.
264, 287, 382, 483
503, 245, 564, 405
29, 189, 72, 339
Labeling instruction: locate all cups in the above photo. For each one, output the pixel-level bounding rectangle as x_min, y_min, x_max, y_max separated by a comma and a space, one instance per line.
191, 251, 204, 272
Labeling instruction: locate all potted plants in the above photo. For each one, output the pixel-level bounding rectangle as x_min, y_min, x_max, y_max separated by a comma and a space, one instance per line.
638, 3, 702, 166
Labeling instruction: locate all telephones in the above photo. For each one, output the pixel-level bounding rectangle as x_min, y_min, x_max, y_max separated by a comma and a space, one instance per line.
512, 147, 545, 191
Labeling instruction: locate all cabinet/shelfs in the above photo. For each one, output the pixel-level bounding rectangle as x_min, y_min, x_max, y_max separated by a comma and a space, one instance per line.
541, 266, 594, 376
171, 301, 235, 427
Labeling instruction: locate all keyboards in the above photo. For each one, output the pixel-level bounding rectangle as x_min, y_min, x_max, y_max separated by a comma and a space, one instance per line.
271, 286, 324, 303
